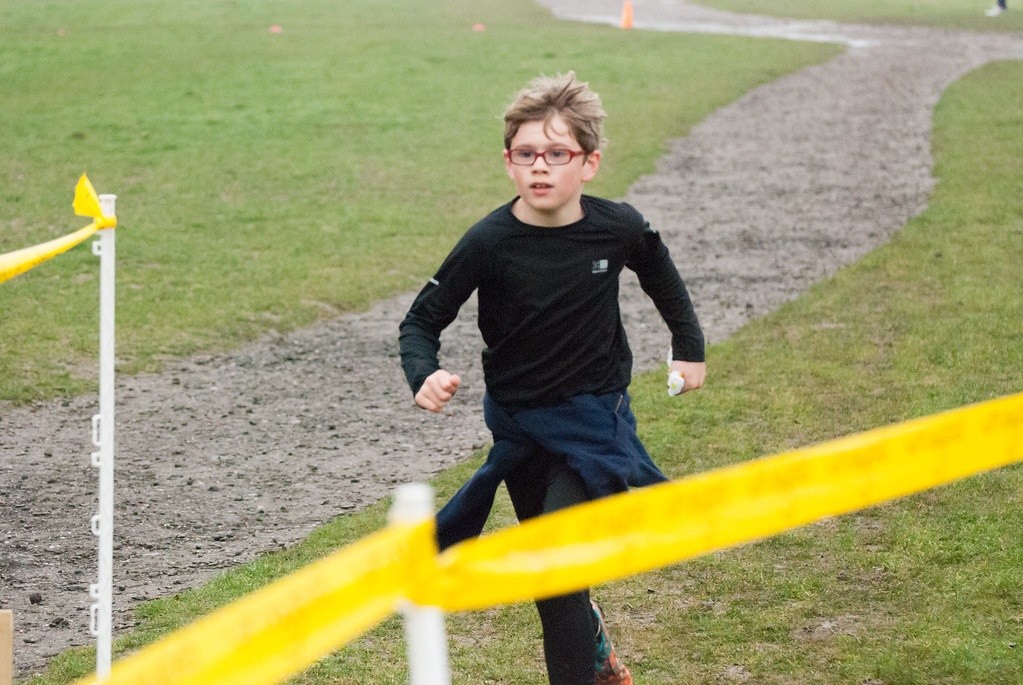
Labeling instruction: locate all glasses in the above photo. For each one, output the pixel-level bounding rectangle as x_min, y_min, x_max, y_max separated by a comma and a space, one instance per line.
507, 147, 585, 166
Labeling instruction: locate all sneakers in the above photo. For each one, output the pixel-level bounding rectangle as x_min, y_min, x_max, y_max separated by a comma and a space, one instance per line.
581, 599, 633, 685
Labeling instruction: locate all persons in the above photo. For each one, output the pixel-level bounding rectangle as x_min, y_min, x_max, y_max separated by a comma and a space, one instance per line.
399, 70, 706, 685
